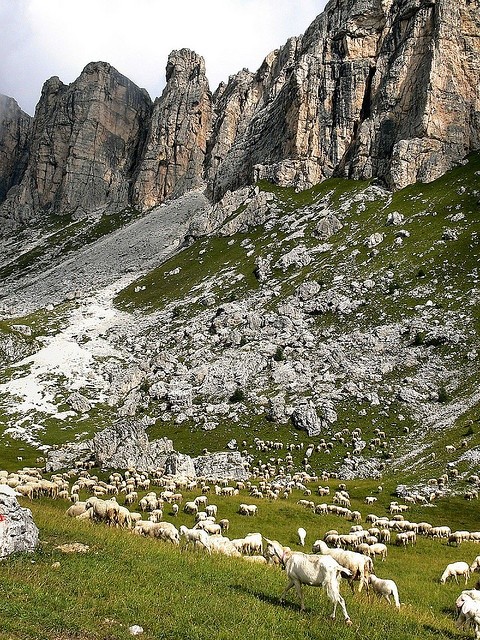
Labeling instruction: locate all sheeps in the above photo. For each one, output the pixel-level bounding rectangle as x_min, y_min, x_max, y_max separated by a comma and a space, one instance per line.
353, 542, 370, 556
445, 446, 456, 453
389, 514, 480, 547
1, 456, 186, 546
239, 503, 258, 517
278, 547, 352, 624
470, 556, 480, 573
311, 540, 373, 593
186, 527, 283, 564
368, 574, 400, 607
297, 527, 306, 547
431, 453, 435, 461
377, 486, 382, 492
184, 496, 230, 534
187, 480, 298, 500
365, 497, 377, 504
404, 426, 409, 433
225, 484, 226, 485
461, 439, 467, 448
438, 561, 470, 586
351, 510, 388, 544
186, 425, 397, 483
298, 483, 351, 517
469, 419, 473, 426
456, 590, 480, 622
371, 543, 388, 561
389, 464, 480, 514
326, 529, 352, 548
